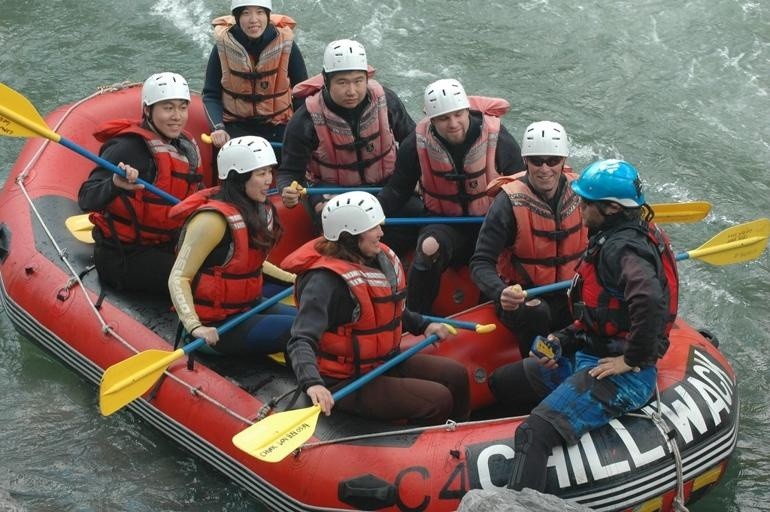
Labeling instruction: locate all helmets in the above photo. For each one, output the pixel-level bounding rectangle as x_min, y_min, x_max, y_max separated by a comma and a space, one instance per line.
423, 78, 471, 120
141, 71, 191, 120
570, 158, 646, 209
217, 135, 279, 181
321, 190, 386, 242
322, 39, 368, 73
230, 0, 273, 12
520, 120, 571, 158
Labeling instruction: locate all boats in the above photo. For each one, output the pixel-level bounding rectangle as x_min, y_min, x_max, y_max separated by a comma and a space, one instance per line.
0, 79, 742, 507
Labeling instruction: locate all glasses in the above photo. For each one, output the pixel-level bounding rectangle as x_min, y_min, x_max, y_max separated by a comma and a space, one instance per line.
523, 154, 566, 168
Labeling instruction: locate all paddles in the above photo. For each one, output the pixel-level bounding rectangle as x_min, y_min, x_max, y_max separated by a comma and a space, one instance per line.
372, 200, 713, 224
514, 217, 770, 300
97, 281, 303, 420
230, 320, 459, 466
65, 185, 282, 246
1, 80, 183, 229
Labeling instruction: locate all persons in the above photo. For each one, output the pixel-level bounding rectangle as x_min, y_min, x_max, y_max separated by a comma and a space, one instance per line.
468, 120, 588, 359
376, 78, 527, 317
201, 0, 308, 186
487, 158, 678, 494
78, 72, 206, 295
274, 39, 424, 260
167, 135, 299, 356
286, 190, 470, 426
